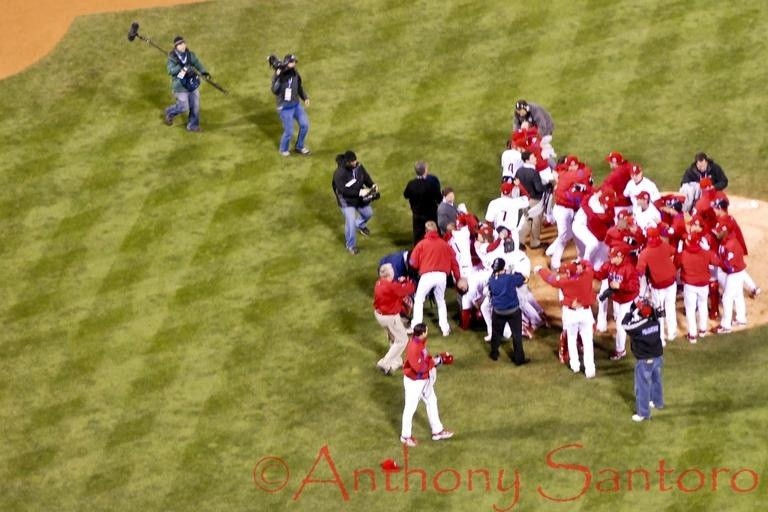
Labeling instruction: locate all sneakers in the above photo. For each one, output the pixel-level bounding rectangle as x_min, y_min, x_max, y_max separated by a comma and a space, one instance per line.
431, 430, 455, 441
710, 325, 732, 334
295, 147, 312, 156
279, 150, 291, 157
609, 350, 627, 361
631, 414, 646, 422
359, 226, 370, 237
399, 436, 418, 447
699, 331, 706, 339
346, 246, 359, 256
686, 333, 698, 344
165, 108, 173, 125
749, 287, 761, 300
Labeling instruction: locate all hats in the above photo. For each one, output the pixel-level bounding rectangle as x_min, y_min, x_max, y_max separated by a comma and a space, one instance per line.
606, 247, 624, 257
605, 151, 624, 165
634, 191, 650, 200
491, 258, 506, 272
282, 53, 297, 65
516, 99, 529, 110
711, 199, 727, 209
476, 225, 493, 235
628, 163, 643, 175
501, 182, 515, 193
700, 178, 713, 190
646, 227, 662, 247
173, 36, 184, 46
685, 231, 701, 248
666, 199, 682, 213
566, 260, 583, 276
599, 190, 616, 209
635, 299, 653, 318
565, 156, 580, 170
718, 213, 735, 233
343, 149, 357, 163
686, 213, 705, 227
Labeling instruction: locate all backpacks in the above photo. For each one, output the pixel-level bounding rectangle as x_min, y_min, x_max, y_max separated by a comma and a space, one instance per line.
169, 48, 200, 91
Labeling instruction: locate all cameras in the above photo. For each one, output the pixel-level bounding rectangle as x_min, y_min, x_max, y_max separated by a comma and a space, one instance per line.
356, 187, 381, 204
269, 56, 296, 82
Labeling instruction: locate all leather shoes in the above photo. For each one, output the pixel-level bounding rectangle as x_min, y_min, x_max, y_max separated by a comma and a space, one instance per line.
516, 357, 531, 366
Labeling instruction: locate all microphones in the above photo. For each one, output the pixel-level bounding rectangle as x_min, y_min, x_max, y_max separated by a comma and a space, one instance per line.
127, 22, 139, 42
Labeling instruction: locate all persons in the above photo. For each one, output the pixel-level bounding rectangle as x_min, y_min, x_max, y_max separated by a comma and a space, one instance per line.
271, 54, 311, 156
632, 191, 661, 238
615, 165, 661, 207
557, 156, 593, 264
331, 151, 380, 255
542, 156, 584, 273
494, 225, 512, 240
516, 152, 549, 250
592, 246, 641, 360
601, 208, 645, 263
691, 178, 729, 227
500, 117, 560, 198
409, 220, 461, 338
437, 186, 461, 237
512, 100, 557, 163
711, 218, 748, 333
572, 188, 615, 268
555, 266, 585, 364
442, 210, 485, 330
164, 37, 211, 132
485, 179, 530, 250
476, 222, 507, 267
400, 325, 454, 447
711, 198, 760, 301
534, 258, 596, 378
373, 263, 416, 376
636, 229, 677, 346
404, 159, 443, 248
487, 259, 530, 366
680, 153, 727, 190
652, 194, 720, 253
497, 239, 547, 329
621, 301, 667, 422
601, 151, 635, 207
673, 232, 722, 343
